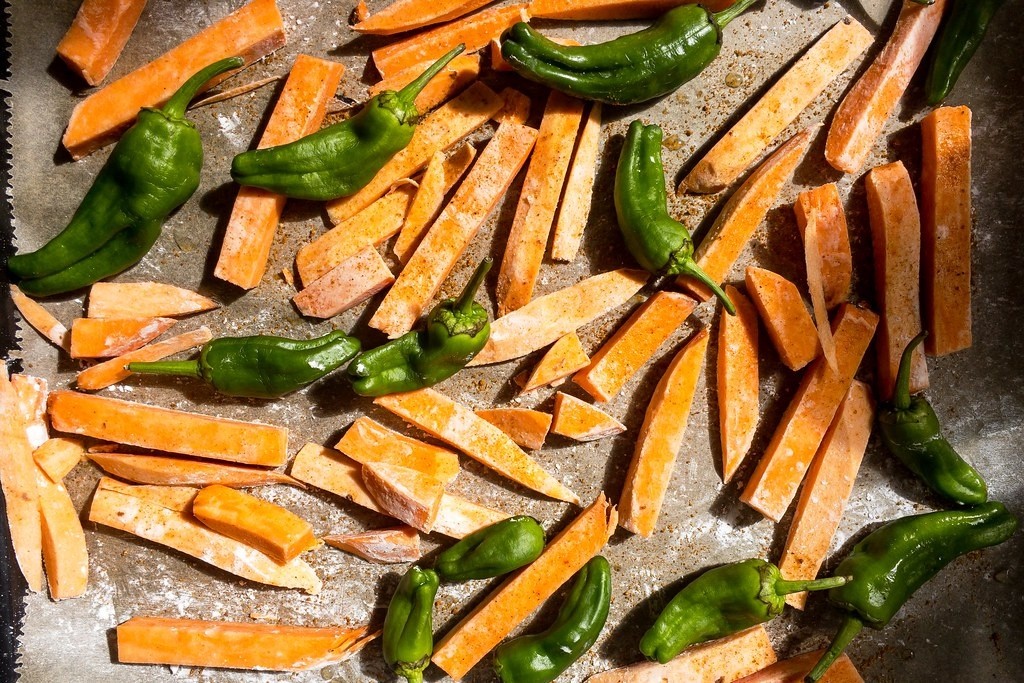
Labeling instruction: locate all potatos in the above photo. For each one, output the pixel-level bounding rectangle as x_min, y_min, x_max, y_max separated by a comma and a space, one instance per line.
1, 0, 975, 683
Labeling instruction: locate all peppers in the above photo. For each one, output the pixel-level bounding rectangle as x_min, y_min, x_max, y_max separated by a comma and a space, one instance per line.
613, 119, 738, 317
923, 0, 1002, 107
4, 56, 244, 299
121, 330, 362, 401
487, 555, 612, 683
437, 515, 548, 582
639, 559, 854, 665
879, 330, 989, 507
345, 256, 495, 397
497, 0, 757, 107
802, 500, 1019, 683
228, 42, 466, 204
383, 564, 440, 683
